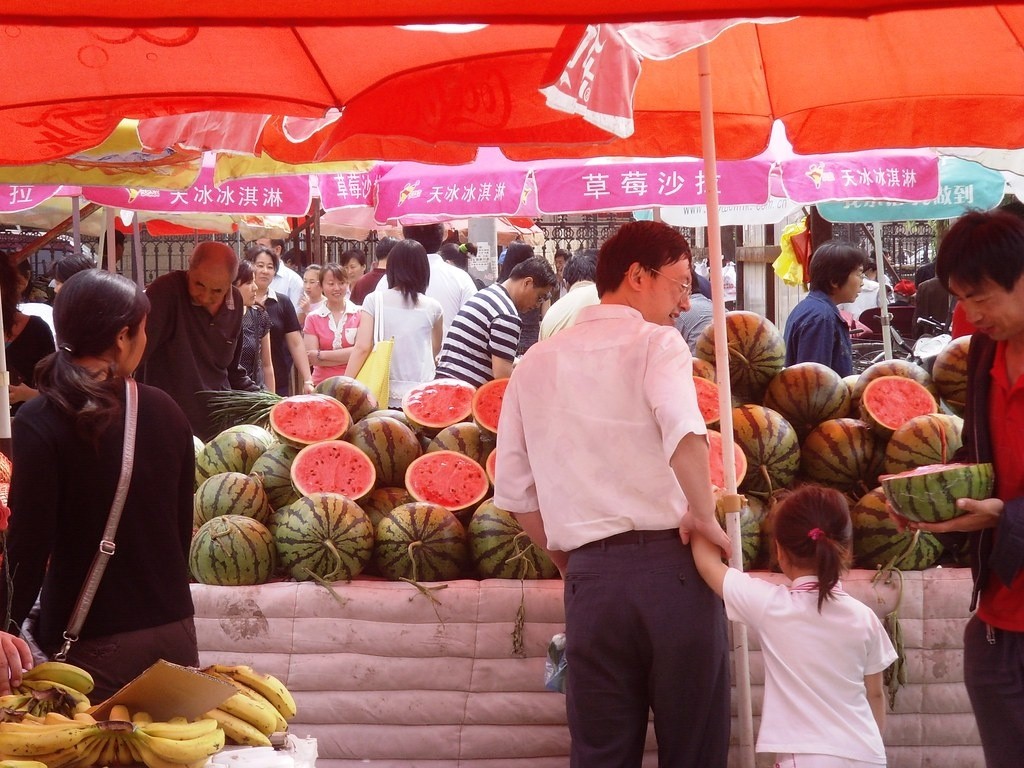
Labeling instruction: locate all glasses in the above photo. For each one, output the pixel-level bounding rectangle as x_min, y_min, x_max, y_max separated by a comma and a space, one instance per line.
625, 266, 692, 304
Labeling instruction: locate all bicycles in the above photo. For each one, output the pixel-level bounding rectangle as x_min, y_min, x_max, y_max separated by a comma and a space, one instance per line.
849, 313, 953, 376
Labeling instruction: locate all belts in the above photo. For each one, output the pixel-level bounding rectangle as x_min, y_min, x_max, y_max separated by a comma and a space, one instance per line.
579, 528, 680, 545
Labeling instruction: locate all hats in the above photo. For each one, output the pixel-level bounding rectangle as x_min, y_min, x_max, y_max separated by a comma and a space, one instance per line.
498, 251, 507, 264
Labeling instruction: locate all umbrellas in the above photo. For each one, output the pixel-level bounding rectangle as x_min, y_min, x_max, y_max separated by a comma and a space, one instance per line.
0, 0, 623, 168
496, 218, 545, 246
136, 0, 1024, 767
0, 152, 1024, 275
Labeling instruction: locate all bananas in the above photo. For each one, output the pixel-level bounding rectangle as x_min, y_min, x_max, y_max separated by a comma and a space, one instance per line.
0, 662, 297, 768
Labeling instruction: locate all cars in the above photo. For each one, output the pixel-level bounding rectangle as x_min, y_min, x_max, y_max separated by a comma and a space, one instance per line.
866, 237, 938, 267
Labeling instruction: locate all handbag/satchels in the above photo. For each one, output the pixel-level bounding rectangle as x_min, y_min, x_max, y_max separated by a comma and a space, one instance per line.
355, 290, 394, 410
19, 599, 47, 668
252, 368, 264, 387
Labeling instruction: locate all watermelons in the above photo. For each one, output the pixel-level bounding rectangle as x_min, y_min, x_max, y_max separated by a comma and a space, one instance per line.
688, 310, 994, 569
184, 375, 559, 583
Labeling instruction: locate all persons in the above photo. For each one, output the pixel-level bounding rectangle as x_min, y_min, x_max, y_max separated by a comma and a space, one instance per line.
784, 239, 880, 378
554, 249, 573, 299
132, 242, 261, 444
493, 219, 732, 768
441, 243, 488, 291
538, 253, 600, 343
233, 238, 444, 398
48, 253, 98, 294
1, 266, 200, 706
687, 485, 901, 768
0, 249, 58, 418
496, 241, 551, 356
434, 256, 554, 389
673, 271, 730, 356
886, 210, 1024, 768
375, 223, 478, 363
915, 254, 938, 289
916, 277, 956, 336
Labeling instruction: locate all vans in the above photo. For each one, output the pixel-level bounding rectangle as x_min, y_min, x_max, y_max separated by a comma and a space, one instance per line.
0, 228, 99, 289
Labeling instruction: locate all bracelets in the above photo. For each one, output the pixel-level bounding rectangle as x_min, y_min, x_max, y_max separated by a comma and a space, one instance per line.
317, 350, 320, 360
304, 380, 314, 386
